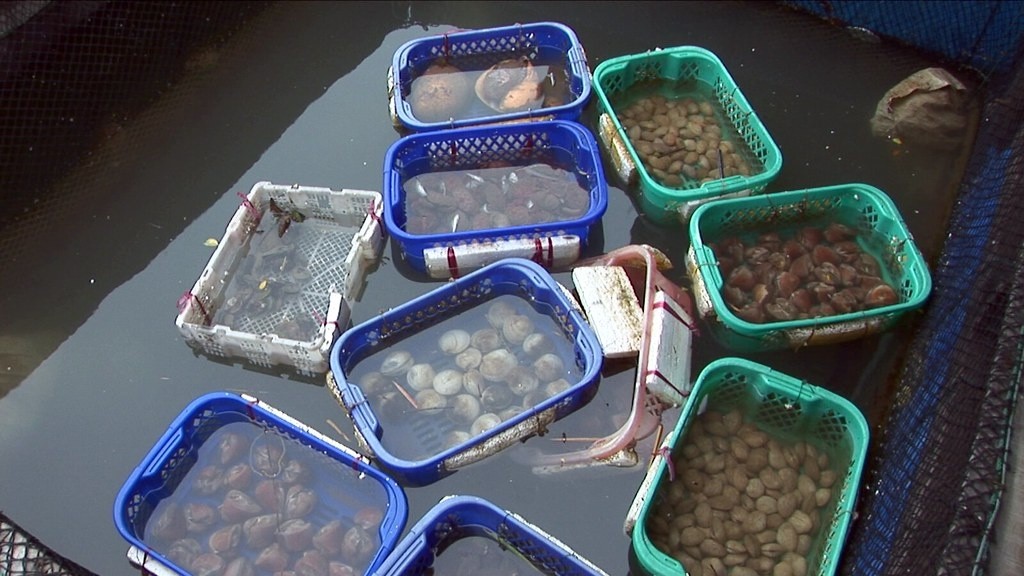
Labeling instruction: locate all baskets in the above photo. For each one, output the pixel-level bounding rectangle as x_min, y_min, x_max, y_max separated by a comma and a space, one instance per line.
371, 495, 609, 576
392, 20, 591, 131
175, 181, 385, 372
329, 257, 602, 482
632, 357, 871, 576
592, 46, 783, 224
114, 391, 408, 576
684, 183, 933, 353
383, 121, 606, 274
